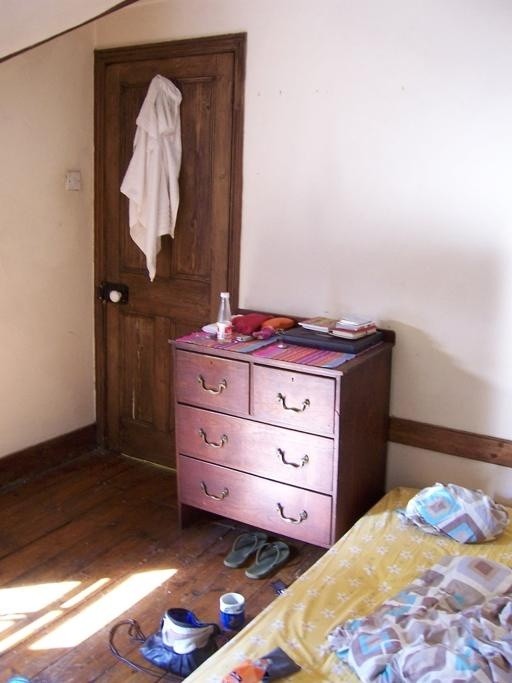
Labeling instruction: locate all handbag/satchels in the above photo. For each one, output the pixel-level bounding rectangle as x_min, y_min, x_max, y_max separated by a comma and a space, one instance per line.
107, 608, 220, 682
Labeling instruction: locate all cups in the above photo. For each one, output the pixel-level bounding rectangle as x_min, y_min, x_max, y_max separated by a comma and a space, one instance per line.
220, 593, 245, 631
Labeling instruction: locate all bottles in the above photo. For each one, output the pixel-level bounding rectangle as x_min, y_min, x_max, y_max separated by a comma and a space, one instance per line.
216, 292, 231, 344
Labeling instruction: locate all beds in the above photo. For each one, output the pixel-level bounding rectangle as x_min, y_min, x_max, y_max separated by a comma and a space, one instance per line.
182, 489, 512, 682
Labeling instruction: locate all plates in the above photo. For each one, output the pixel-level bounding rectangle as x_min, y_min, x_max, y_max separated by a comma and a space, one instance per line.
202, 323, 216, 337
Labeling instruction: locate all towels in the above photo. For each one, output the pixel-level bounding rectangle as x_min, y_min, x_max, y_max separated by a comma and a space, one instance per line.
118, 74, 181, 283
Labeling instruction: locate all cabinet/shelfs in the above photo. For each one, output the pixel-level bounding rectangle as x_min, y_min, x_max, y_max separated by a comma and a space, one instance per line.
167, 309, 395, 548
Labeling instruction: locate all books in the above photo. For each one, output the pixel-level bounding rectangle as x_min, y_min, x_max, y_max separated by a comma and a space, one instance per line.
280, 313, 385, 353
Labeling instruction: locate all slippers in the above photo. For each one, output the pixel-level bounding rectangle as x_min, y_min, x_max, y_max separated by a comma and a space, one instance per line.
224, 532, 290, 579
